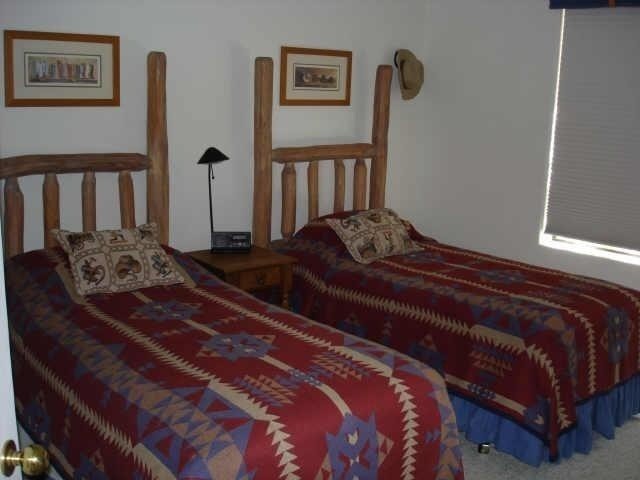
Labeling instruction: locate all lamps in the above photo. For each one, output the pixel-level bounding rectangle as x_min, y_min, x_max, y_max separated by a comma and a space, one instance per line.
198, 147, 251, 254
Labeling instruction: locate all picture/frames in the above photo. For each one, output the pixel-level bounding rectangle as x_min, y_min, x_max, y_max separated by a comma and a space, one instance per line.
3, 29, 121, 106
280, 46, 352, 106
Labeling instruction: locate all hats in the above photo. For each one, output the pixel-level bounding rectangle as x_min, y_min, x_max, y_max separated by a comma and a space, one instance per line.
394, 49, 424, 100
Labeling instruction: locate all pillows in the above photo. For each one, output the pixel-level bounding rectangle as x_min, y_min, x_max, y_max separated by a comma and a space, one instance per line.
50, 222, 186, 297
325, 206, 425, 265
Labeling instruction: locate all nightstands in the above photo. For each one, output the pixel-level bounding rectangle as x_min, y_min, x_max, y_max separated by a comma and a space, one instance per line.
186, 243, 299, 307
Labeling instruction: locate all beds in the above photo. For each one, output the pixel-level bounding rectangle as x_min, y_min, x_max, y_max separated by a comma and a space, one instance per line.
253, 57, 639, 464
0, 49, 468, 479
